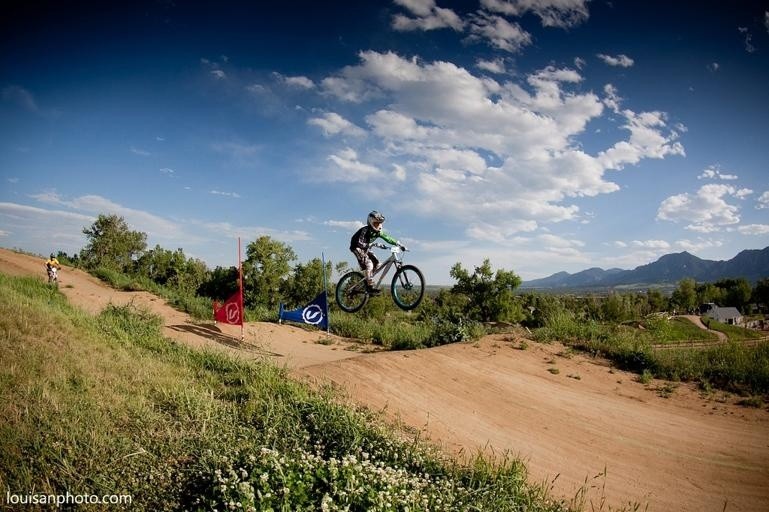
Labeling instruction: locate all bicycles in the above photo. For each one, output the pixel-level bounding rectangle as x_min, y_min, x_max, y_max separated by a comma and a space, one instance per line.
333, 241, 426, 314
48, 267, 61, 288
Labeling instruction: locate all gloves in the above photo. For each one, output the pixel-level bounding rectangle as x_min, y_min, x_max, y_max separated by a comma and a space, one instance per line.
381, 244, 389, 249
400, 245, 407, 251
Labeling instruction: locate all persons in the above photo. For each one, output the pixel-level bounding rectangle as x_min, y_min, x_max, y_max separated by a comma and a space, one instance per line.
350, 210, 406, 294
45, 252, 62, 271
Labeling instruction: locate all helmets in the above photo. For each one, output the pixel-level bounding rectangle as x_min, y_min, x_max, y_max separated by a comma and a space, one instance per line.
367, 210, 385, 231
50, 252, 55, 257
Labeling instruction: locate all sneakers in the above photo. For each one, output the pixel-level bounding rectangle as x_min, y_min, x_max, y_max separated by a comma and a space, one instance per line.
365, 285, 381, 295
